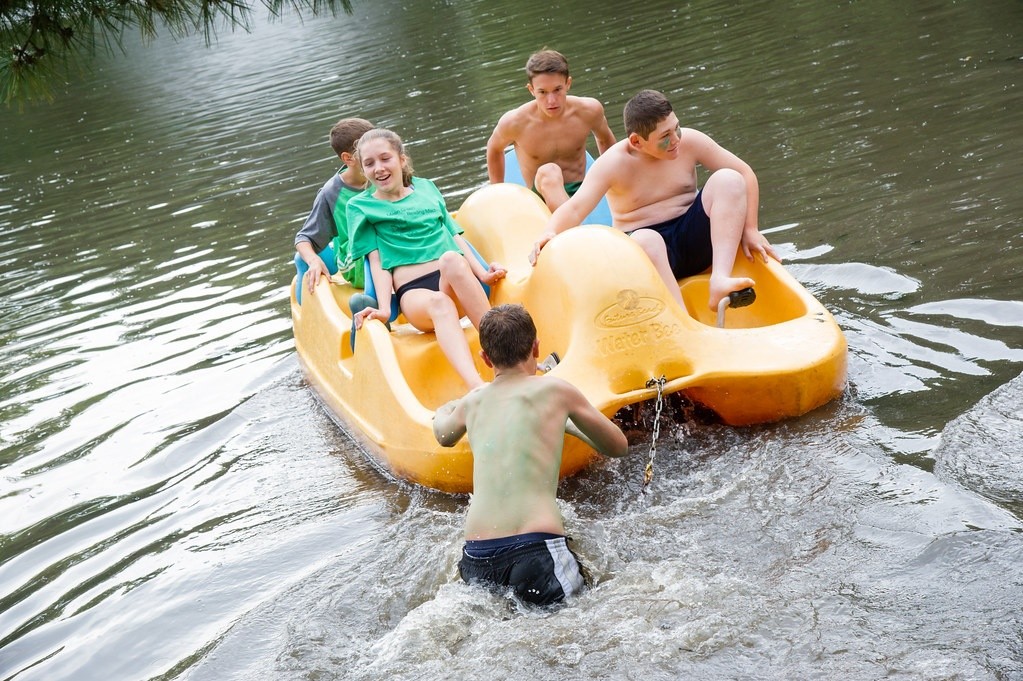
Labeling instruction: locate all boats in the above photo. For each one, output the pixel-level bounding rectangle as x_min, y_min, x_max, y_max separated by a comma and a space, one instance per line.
286, 143, 851, 498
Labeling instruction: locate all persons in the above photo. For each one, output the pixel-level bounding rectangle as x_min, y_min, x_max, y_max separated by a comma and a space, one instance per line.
528, 89, 783, 316
430, 303, 628, 612
346, 128, 510, 394
486, 48, 617, 216
293, 116, 376, 296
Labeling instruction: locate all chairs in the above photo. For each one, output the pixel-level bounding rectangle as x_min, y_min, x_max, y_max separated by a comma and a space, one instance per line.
583, 195, 613, 229
294, 241, 340, 305
363, 235, 492, 322
503, 144, 597, 188
